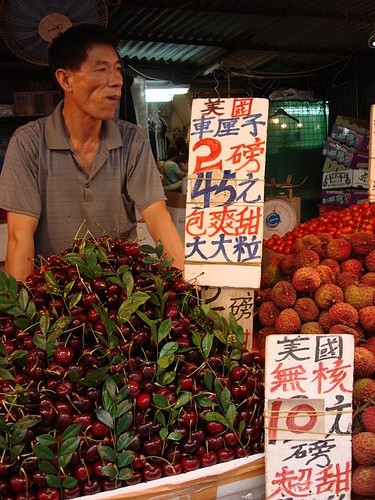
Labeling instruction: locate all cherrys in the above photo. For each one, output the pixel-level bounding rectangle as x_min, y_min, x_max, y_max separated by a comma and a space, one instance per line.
0, 234, 263, 500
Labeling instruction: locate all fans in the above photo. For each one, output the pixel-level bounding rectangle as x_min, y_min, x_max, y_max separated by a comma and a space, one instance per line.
0, 0, 109, 66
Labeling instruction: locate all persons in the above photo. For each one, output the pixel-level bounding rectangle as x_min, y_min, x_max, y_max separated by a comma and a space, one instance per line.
163, 146, 184, 186
168, 138, 188, 164
1, 25, 188, 287
162, 155, 187, 193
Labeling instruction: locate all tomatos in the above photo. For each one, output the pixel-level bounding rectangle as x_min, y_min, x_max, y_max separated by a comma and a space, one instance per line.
262, 201, 375, 255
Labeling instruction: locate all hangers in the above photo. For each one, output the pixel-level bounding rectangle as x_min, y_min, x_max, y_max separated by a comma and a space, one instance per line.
269, 108, 299, 122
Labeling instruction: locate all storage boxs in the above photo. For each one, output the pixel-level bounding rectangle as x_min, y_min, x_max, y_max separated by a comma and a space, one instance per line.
63, 451, 267, 500
11, 90, 65, 117
